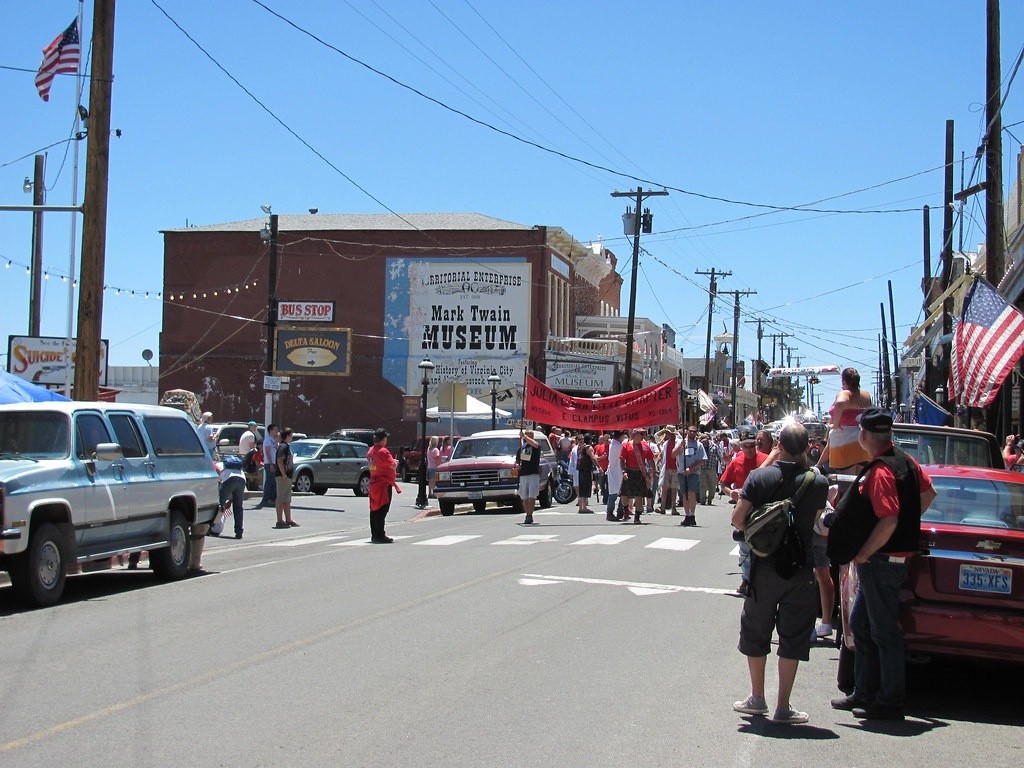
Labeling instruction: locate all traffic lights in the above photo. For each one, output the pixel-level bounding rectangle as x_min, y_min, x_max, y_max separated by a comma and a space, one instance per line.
810, 379, 818, 384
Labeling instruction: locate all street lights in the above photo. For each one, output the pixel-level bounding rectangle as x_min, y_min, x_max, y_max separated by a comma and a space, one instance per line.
415, 354, 435, 509
260, 204, 281, 443
487, 371, 501, 431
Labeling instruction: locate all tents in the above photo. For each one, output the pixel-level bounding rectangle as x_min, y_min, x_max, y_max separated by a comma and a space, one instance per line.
426, 395, 512, 422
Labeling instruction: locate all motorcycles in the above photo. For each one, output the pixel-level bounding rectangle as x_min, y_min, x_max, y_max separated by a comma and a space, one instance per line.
555, 459, 575, 505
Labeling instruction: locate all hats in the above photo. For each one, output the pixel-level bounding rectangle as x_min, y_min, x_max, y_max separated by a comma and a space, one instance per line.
738, 430, 756, 444
247, 421, 256, 427
855, 406, 895, 432
371, 427, 392, 440
662, 425, 679, 436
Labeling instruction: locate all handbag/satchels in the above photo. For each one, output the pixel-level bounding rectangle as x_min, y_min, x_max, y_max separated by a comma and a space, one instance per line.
741, 499, 797, 559
645, 474, 653, 490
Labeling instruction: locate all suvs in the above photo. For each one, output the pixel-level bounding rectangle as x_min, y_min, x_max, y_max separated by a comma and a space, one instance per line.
433, 429, 558, 516
400, 436, 464, 483
210, 422, 374, 496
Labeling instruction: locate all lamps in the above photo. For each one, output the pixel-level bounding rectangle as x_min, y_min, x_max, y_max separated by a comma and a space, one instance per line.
142, 349, 153, 366
23, 176, 34, 193
261, 205, 272, 215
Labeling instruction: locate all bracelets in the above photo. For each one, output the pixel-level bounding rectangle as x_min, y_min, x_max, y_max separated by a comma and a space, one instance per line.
1006, 444, 1011, 446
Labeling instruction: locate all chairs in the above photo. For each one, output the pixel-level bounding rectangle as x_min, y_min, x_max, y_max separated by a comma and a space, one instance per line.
1000, 505, 1024, 529
921, 510, 944, 520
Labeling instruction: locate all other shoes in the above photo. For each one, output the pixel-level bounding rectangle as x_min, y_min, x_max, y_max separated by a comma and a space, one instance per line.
851, 707, 907, 720
829, 693, 869, 710
606, 514, 620, 522
707, 501, 712, 506
286, 520, 301, 527
633, 517, 642, 524
648, 507, 654, 513
524, 515, 534, 524
688, 515, 697, 526
579, 509, 595, 513
680, 515, 689, 526
234, 534, 243, 539
729, 499, 737, 504
622, 512, 630, 521
276, 520, 292, 528
701, 501, 705, 505
655, 507, 666, 514
816, 622, 833, 638
672, 509, 680, 515
370, 535, 394, 544
616, 512, 631, 519
810, 629, 820, 643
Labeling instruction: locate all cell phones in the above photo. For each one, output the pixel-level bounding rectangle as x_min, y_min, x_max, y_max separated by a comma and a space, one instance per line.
1014, 434, 1020, 445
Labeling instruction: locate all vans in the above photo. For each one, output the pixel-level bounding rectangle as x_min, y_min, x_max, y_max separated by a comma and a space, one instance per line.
0, 401, 222, 607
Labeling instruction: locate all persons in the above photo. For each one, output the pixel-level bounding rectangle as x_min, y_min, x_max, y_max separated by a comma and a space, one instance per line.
198, 411, 217, 460
239, 422, 263, 490
427, 436, 451, 498
516, 429, 541, 526
818, 368, 871, 467
814, 486, 838, 636
205, 461, 247, 539
262, 424, 300, 527
720, 430, 768, 593
1002, 435, 1024, 473
366, 428, 399, 543
729, 422, 829, 724
536, 417, 826, 525
825, 407, 938, 722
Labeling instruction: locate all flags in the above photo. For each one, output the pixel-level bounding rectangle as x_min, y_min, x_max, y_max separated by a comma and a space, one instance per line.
224, 509, 232, 518
35, 15, 80, 102
947, 277, 1024, 408
919, 394, 948, 449
893, 412, 903, 422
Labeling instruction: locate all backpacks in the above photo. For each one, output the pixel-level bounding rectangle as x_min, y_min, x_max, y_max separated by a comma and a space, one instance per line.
224, 454, 244, 469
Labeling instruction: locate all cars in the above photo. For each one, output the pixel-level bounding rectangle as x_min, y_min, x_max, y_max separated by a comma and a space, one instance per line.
736, 412, 827, 439
838, 463, 1024, 695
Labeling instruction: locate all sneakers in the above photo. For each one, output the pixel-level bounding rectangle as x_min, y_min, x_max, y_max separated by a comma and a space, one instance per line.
733, 694, 770, 715
772, 703, 812, 723
